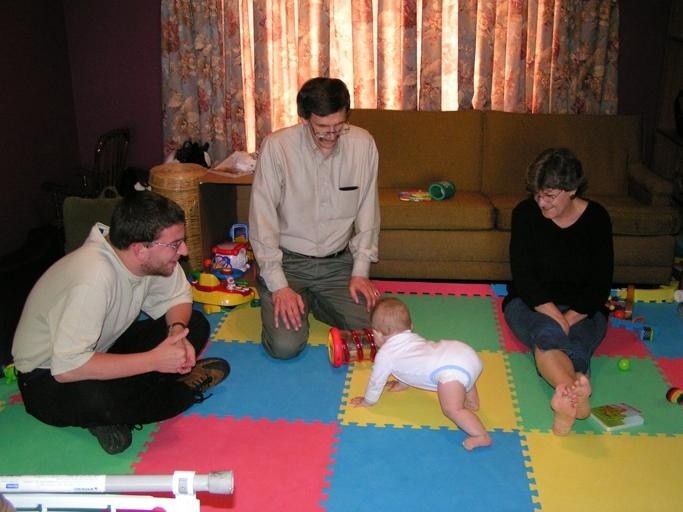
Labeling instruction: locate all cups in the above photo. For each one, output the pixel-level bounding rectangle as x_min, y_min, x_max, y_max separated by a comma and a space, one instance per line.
428, 180, 455, 201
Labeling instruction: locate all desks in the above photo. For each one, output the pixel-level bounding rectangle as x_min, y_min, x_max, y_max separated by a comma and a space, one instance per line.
199, 161, 256, 273
42, 164, 150, 227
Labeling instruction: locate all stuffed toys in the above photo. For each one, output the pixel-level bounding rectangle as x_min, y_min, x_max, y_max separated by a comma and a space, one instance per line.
117, 167, 152, 197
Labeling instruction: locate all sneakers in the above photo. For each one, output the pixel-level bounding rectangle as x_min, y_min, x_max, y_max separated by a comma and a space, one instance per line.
87, 425, 144, 455
162, 358, 230, 403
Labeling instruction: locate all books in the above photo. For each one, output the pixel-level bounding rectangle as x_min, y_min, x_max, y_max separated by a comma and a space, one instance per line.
591, 402, 644, 431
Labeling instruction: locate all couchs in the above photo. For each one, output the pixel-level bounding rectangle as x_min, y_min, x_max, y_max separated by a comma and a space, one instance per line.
347, 107, 681, 288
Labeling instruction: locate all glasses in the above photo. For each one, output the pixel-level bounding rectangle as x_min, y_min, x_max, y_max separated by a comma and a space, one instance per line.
534, 190, 563, 201
148, 241, 182, 250
307, 119, 350, 137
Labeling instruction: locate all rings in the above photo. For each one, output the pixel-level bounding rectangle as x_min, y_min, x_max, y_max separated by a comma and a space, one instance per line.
375, 293, 378, 296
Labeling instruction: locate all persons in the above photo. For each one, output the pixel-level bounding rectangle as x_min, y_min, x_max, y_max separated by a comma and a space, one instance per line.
11, 189, 230, 455
349, 298, 492, 451
502, 147, 614, 435
248, 77, 382, 360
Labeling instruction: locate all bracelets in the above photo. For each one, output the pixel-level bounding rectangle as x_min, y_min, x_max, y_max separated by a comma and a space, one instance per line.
168, 322, 185, 330
